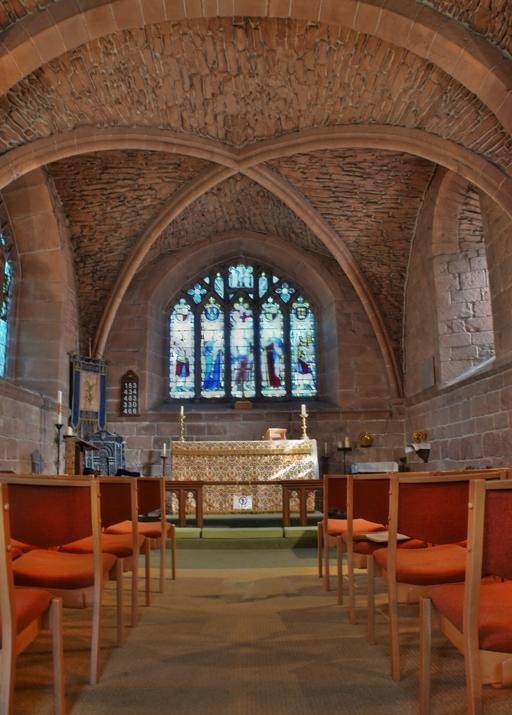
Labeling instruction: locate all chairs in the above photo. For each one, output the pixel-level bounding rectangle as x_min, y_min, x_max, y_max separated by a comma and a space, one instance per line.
366, 476, 471, 683
0, 483, 66, 714
317, 476, 350, 590
419, 479, 512, 715
104, 476, 176, 593
58, 478, 150, 624
337, 474, 395, 624
2, 474, 123, 685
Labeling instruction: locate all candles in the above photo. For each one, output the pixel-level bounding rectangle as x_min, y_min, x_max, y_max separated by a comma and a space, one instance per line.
161, 443, 167, 457
179, 406, 184, 416
301, 404, 306, 415
323, 441, 328, 456
56, 391, 63, 424
